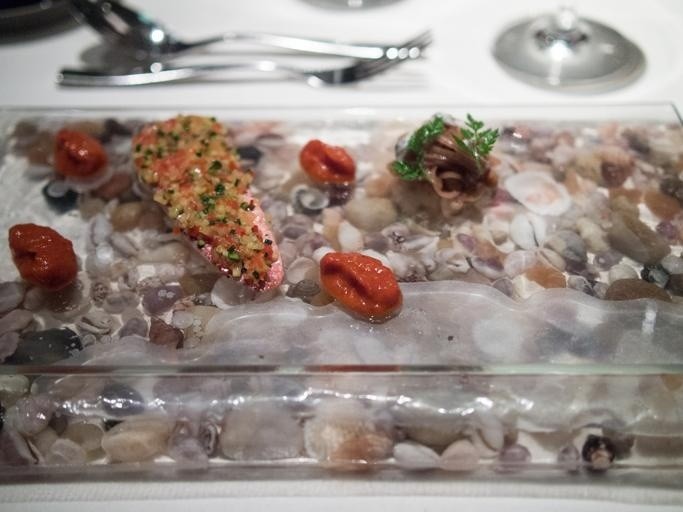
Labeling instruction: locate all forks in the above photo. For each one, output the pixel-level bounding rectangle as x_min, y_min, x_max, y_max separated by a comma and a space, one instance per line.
69, 0, 424, 62
53, 35, 438, 99
